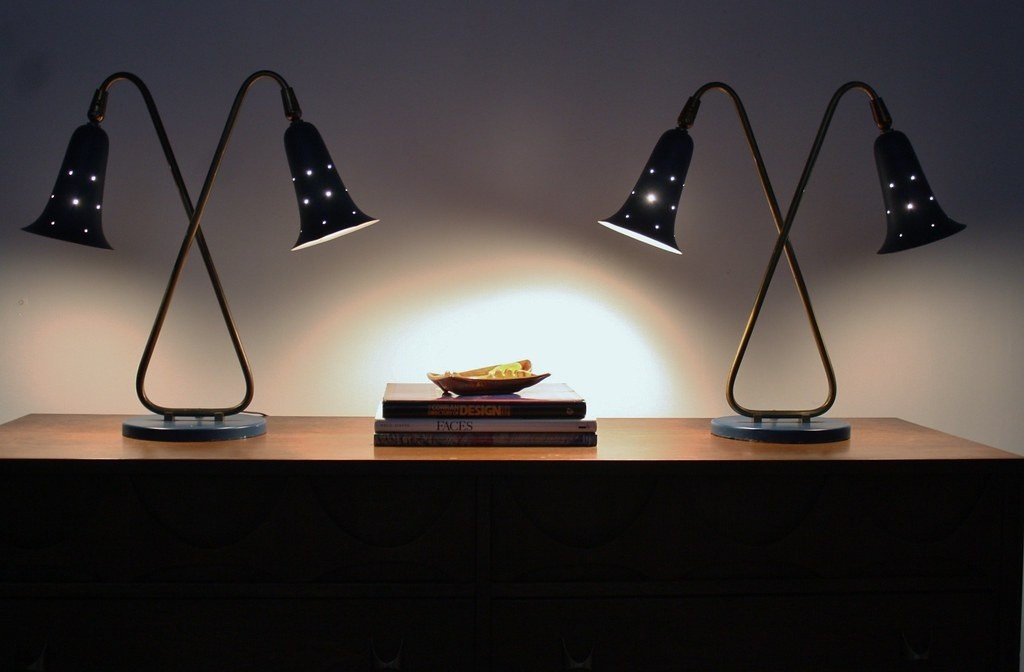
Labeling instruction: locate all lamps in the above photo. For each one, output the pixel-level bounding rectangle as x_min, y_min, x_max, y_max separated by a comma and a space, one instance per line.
599, 81, 965, 445
21, 70, 380, 441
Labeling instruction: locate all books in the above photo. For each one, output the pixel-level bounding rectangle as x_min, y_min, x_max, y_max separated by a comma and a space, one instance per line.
373, 383, 597, 448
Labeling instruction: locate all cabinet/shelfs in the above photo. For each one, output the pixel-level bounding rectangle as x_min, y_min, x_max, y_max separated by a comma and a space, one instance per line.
0, 413, 1024, 672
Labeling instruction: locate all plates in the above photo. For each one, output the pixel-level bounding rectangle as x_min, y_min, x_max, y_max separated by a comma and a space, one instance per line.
427, 361, 550, 395
